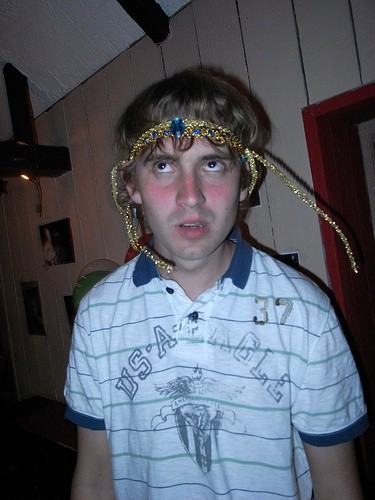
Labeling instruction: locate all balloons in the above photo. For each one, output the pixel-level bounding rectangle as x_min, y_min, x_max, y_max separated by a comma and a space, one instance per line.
71, 270, 111, 309
124, 234, 152, 264
77, 259, 120, 281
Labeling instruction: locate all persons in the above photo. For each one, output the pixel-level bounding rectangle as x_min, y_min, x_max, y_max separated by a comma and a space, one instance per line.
41, 227, 58, 266
62, 68, 369, 500
